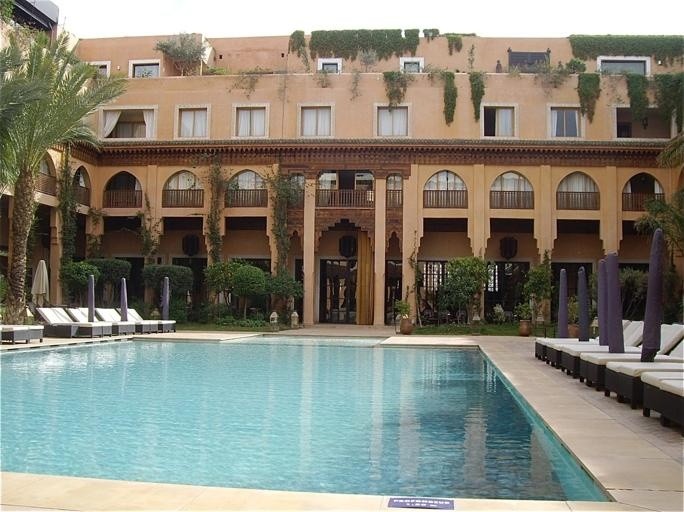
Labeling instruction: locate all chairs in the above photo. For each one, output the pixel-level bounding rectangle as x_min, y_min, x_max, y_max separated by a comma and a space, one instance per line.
534, 315, 684, 432
0, 307, 178, 345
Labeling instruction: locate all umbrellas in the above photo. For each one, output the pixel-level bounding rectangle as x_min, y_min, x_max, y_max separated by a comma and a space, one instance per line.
31, 259, 49, 308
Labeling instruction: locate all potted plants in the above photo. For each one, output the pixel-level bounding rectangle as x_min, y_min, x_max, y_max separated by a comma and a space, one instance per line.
566, 296, 580, 337
394, 300, 414, 334
515, 303, 532, 337
